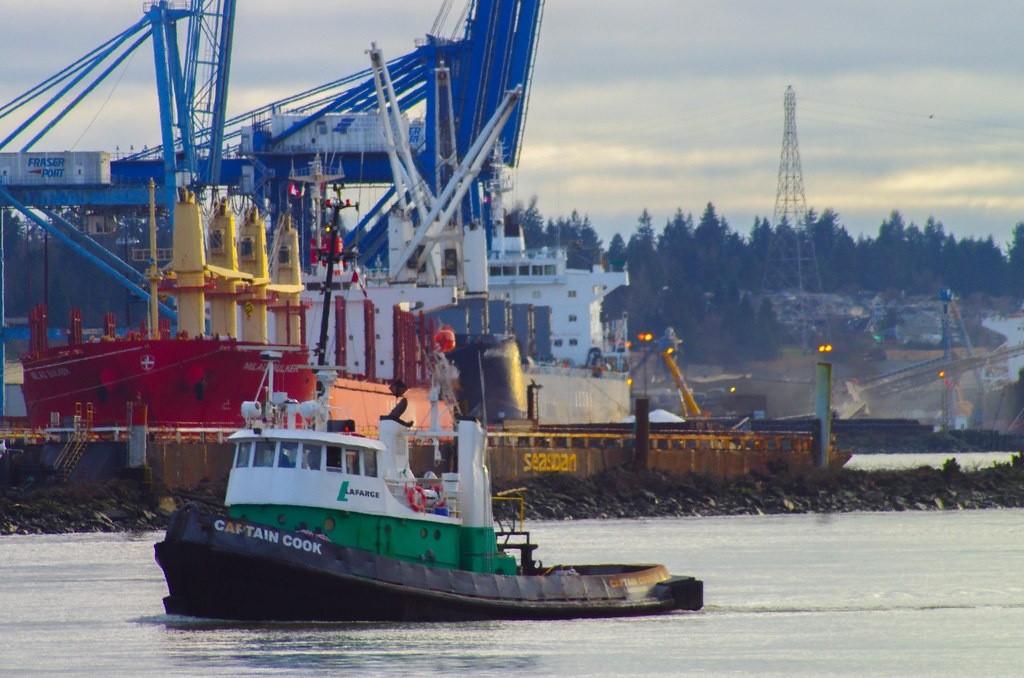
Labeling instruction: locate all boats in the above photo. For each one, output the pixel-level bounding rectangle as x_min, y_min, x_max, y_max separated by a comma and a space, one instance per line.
151, 182, 706, 624
19, 190, 455, 442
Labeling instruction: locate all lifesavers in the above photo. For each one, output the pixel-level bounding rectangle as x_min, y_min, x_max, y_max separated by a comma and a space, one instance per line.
408, 485, 427, 511
430, 484, 447, 506
